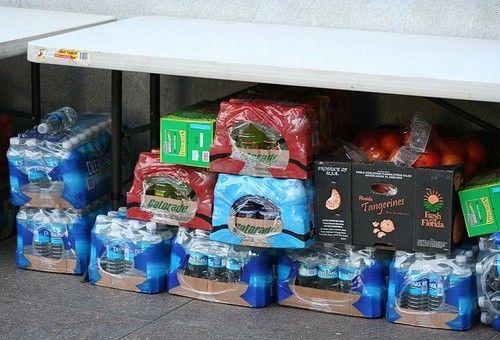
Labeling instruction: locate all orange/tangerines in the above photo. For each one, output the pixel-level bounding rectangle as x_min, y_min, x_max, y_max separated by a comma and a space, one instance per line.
355, 127, 484, 193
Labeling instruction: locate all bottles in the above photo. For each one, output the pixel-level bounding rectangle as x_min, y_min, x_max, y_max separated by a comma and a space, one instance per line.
395, 232, 500, 333
6, 106, 377, 293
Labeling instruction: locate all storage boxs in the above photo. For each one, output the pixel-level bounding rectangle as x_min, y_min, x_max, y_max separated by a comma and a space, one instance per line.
312, 143, 467, 255
208, 143, 326, 180
167, 271, 276, 308
126, 191, 214, 231
209, 215, 316, 249
160, 99, 222, 168
386, 304, 479, 331
9, 175, 112, 208
15, 250, 90, 276
88, 267, 169, 294
457, 163, 500, 238
277, 281, 388, 319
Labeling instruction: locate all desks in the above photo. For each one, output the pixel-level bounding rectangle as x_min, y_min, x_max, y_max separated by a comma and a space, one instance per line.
0, 6, 500, 244
